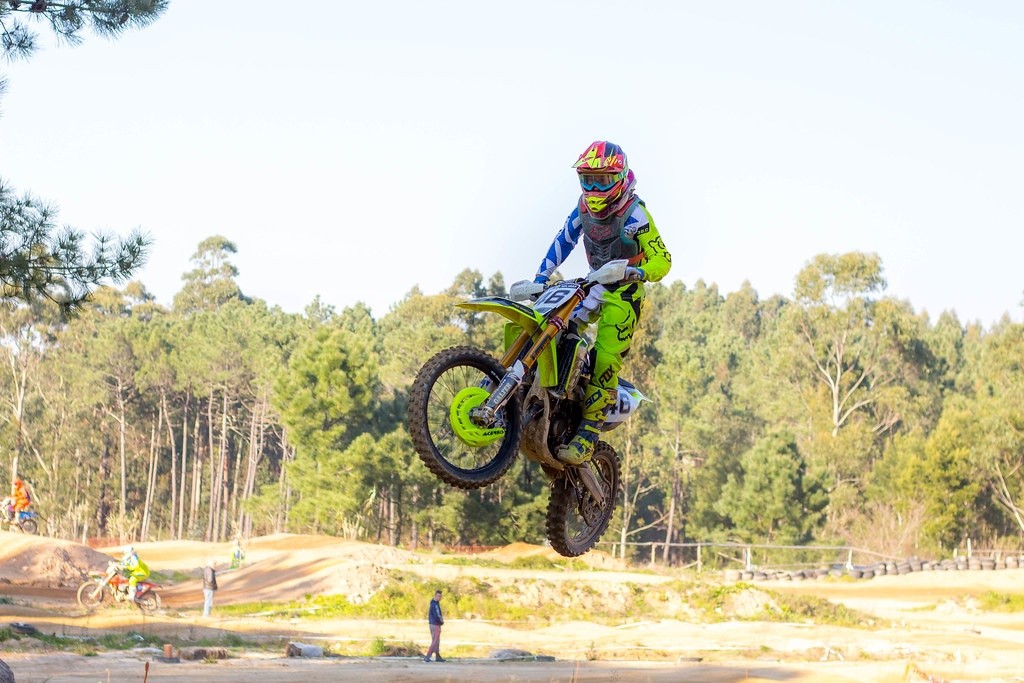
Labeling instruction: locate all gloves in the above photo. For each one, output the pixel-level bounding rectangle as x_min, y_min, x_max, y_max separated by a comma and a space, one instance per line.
607, 265, 643, 281
529, 276, 550, 302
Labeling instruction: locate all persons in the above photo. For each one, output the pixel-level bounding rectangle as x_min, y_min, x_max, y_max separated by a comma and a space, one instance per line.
201, 557, 219, 621
425, 588, 447, 665
529, 139, 673, 466
4, 479, 31, 525
119, 544, 150, 602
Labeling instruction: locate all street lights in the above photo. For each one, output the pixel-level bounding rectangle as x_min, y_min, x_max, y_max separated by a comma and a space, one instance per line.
647, 505, 671, 567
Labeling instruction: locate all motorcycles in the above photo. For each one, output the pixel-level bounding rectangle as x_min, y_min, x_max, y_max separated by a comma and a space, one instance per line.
408, 260, 631, 557
77, 561, 162, 614
0, 497, 39, 534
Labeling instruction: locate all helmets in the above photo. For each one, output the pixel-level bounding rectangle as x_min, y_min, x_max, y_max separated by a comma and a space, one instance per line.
14, 479, 22, 485
123, 545, 132, 554
572, 141, 629, 213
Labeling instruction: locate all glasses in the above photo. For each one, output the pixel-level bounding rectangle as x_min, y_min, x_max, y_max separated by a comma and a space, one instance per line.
577, 164, 628, 191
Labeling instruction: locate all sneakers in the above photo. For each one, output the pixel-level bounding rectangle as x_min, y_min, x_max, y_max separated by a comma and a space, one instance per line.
422, 657, 432, 663
435, 658, 446, 663
124, 595, 134, 602
11, 521, 19, 524
558, 422, 601, 464
